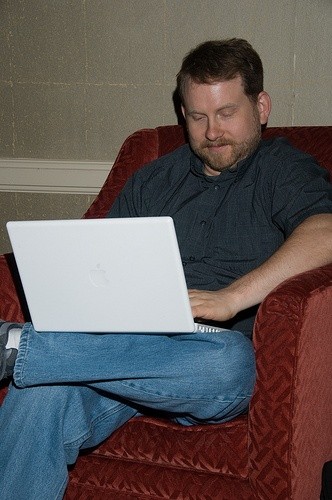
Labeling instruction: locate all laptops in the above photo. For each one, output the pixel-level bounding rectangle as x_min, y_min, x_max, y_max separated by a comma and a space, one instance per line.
6, 216, 231, 334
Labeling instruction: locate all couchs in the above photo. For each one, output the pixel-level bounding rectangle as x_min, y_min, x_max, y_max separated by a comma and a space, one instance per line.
0, 126, 332, 500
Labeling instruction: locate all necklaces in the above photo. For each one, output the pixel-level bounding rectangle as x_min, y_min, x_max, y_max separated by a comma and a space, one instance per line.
1, 38, 332, 500
